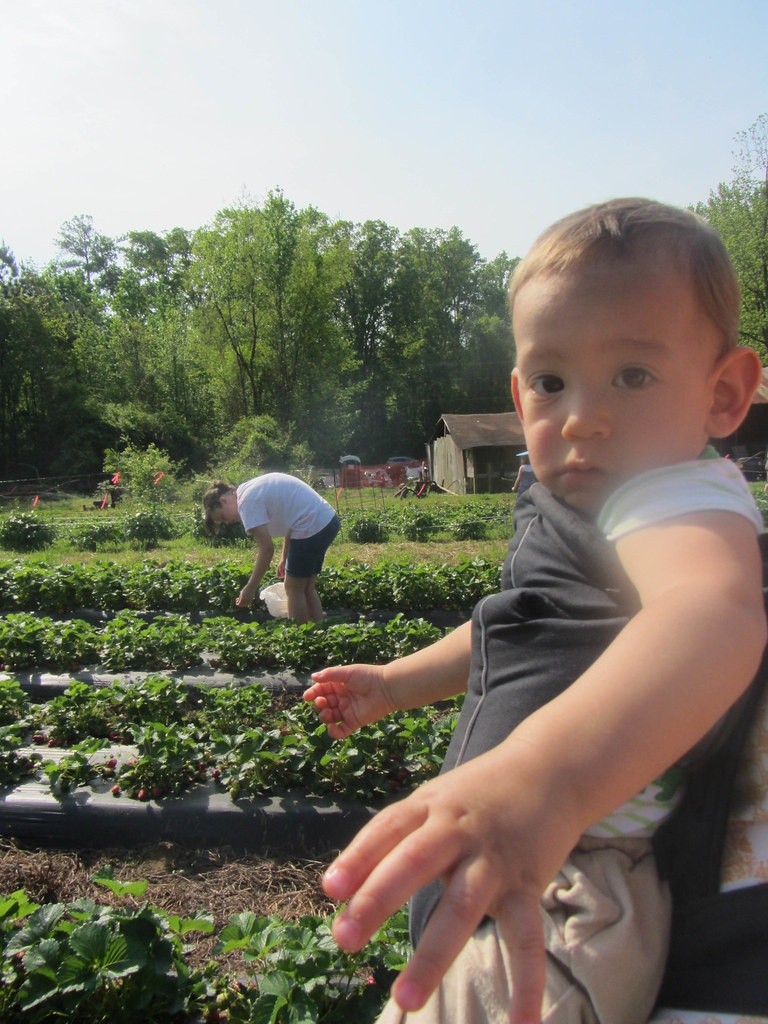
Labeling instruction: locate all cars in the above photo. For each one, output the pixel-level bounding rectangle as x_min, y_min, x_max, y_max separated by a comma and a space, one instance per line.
386, 457, 419, 465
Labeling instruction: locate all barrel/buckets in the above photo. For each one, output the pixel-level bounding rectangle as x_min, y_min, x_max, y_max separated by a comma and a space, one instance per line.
265, 577, 288, 618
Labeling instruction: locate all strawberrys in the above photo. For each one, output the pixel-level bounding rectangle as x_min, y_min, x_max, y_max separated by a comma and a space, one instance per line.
104, 759, 243, 801
32, 735, 55, 747
108, 731, 133, 744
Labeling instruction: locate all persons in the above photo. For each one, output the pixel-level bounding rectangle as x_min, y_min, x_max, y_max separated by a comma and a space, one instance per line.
303, 196, 768, 1024
203, 473, 341, 620
